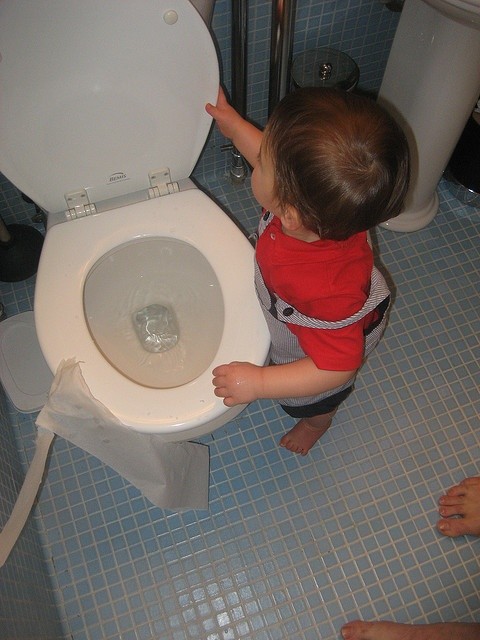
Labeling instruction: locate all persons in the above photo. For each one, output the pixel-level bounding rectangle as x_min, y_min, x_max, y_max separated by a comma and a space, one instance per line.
337, 475, 480, 640
202, 83, 413, 458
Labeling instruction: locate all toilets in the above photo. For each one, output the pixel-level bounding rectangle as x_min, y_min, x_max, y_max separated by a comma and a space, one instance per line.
0, 0, 273, 441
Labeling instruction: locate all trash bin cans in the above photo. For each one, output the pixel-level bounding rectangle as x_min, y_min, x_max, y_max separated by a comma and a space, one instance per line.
0, 311, 59, 415
